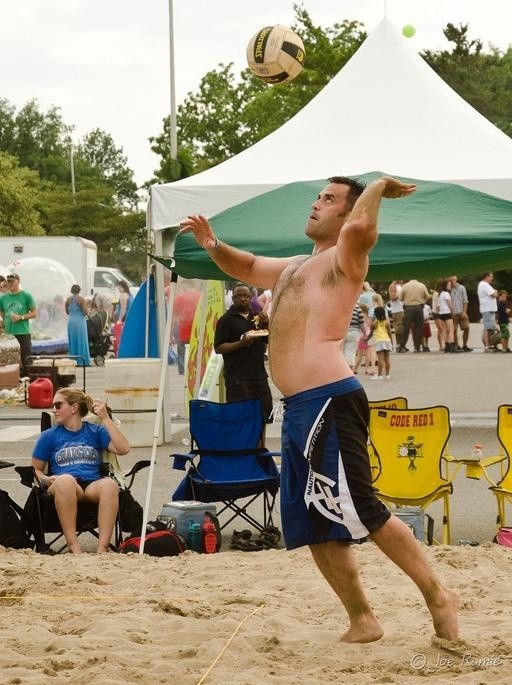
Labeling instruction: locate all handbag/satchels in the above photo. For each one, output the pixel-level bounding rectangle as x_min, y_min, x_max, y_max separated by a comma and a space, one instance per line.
118, 520, 187, 557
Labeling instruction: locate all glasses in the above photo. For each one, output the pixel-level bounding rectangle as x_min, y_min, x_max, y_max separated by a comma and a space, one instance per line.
52, 401, 66, 409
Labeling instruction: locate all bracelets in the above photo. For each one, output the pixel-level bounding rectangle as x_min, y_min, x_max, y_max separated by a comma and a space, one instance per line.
214, 236, 220, 249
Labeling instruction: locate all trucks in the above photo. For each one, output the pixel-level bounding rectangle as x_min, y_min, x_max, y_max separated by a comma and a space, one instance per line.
0, 232, 142, 315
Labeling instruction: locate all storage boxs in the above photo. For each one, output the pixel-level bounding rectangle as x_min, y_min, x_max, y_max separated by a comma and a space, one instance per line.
390, 508, 424, 543
163, 500, 217, 547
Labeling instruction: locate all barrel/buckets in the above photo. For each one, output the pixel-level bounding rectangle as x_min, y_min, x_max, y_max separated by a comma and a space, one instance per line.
27, 376, 55, 408
101, 357, 163, 447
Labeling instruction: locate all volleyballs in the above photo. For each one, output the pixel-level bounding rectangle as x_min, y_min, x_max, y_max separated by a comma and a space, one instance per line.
246, 26, 305, 85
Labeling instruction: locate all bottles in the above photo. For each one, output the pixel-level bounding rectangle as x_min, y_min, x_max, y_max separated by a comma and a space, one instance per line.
187, 522, 202, 554
472, 444, 482, 462
201, 515, 220, 554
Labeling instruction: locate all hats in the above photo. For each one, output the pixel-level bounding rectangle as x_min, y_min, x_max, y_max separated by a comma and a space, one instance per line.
8, 273, 19, 281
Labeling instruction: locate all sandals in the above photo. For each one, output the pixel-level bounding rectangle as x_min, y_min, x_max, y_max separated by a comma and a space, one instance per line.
230, 526, 281, 552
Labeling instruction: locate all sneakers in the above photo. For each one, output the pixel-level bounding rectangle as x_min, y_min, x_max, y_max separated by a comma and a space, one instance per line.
396, 345, 430, 353
438, 341, 472, 352
370, 374, 390, 380
486, 345, 511, 353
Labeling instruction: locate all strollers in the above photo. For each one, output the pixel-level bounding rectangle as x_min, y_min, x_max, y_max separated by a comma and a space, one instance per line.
81, 307, 113, 367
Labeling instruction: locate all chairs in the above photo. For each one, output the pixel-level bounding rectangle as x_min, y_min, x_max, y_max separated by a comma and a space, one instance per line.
462, 404, 512, 545
15, 409, 150, 553
168, 399, 282, 546
369, 397, 408, 482
370, 408, 478, 546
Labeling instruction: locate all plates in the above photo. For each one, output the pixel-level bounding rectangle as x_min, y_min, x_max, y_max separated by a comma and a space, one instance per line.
247, 332, 269, 337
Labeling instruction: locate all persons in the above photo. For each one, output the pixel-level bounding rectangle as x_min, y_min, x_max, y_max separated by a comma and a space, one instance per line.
224, 280, 272, 322
176, 174, 459, 646
214, 285, 273, 449
342, 272, 511, 380
30, 388, 130, 554
156, 272, 204, 376
0, 272, 134, 379
